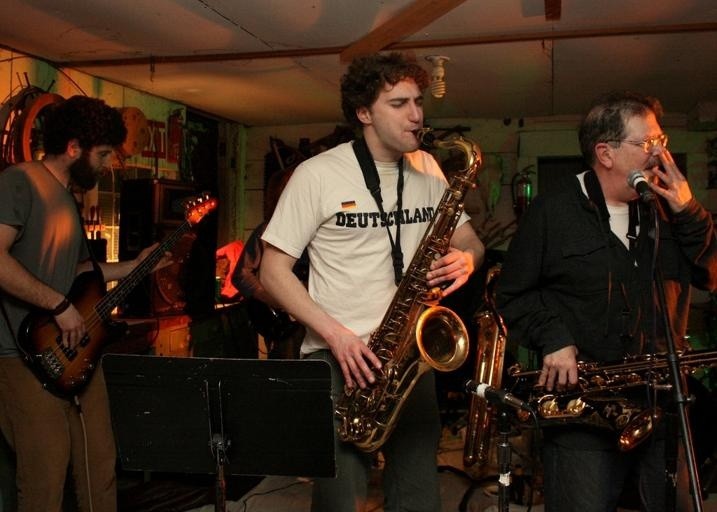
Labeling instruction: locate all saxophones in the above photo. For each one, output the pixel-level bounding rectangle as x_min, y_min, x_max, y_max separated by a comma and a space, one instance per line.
506, 346, 717, 452
333, 126, 484, 454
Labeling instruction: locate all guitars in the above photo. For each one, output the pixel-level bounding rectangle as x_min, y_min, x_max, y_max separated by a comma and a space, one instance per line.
15, 192, 216, 401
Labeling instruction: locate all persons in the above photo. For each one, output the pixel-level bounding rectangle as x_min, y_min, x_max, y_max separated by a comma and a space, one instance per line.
0, 95, 122, 510
493, 88, 717, 512
255, 49, 490, 510
230, 216, 309, 358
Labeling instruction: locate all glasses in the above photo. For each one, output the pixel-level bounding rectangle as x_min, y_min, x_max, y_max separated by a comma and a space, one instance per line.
605, 134, 668, 152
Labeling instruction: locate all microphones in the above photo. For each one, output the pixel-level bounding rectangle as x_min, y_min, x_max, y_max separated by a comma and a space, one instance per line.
627, 169, 657, 209
463, 378, 529, 411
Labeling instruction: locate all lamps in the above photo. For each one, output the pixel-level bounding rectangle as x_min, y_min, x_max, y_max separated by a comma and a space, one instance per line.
150, 64, 158, 83
425, 55, 450, 98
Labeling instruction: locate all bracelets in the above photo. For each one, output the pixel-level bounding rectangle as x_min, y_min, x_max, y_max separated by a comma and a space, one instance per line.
51, 296, 70, 316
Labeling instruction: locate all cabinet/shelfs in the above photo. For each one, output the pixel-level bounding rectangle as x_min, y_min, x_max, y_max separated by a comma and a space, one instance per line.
111, 314, 193, 358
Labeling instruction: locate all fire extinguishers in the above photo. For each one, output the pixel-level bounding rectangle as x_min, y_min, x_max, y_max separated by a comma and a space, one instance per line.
511, 165, 536, 210
167, 108, 184, 162
